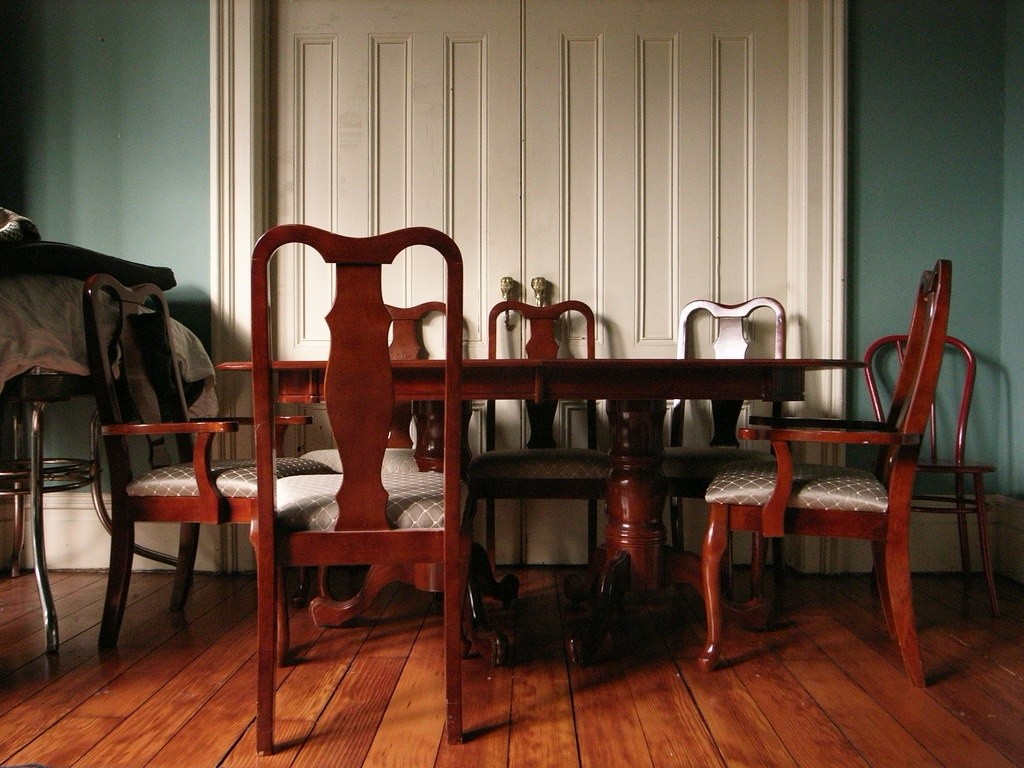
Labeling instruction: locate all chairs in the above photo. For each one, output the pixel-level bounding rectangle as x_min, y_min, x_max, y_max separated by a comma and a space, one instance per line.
694, 257, 957, 691
79, 275, 337, 668
254, 224, 468, 755
484, 300, 613, 581
667, 295, 808, 620
325, 298, 469, 603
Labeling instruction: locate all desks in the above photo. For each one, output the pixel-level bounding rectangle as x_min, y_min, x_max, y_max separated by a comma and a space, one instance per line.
863, 335, 1003, 618
206, 355, 868, 663
1, 369, 174, 663
